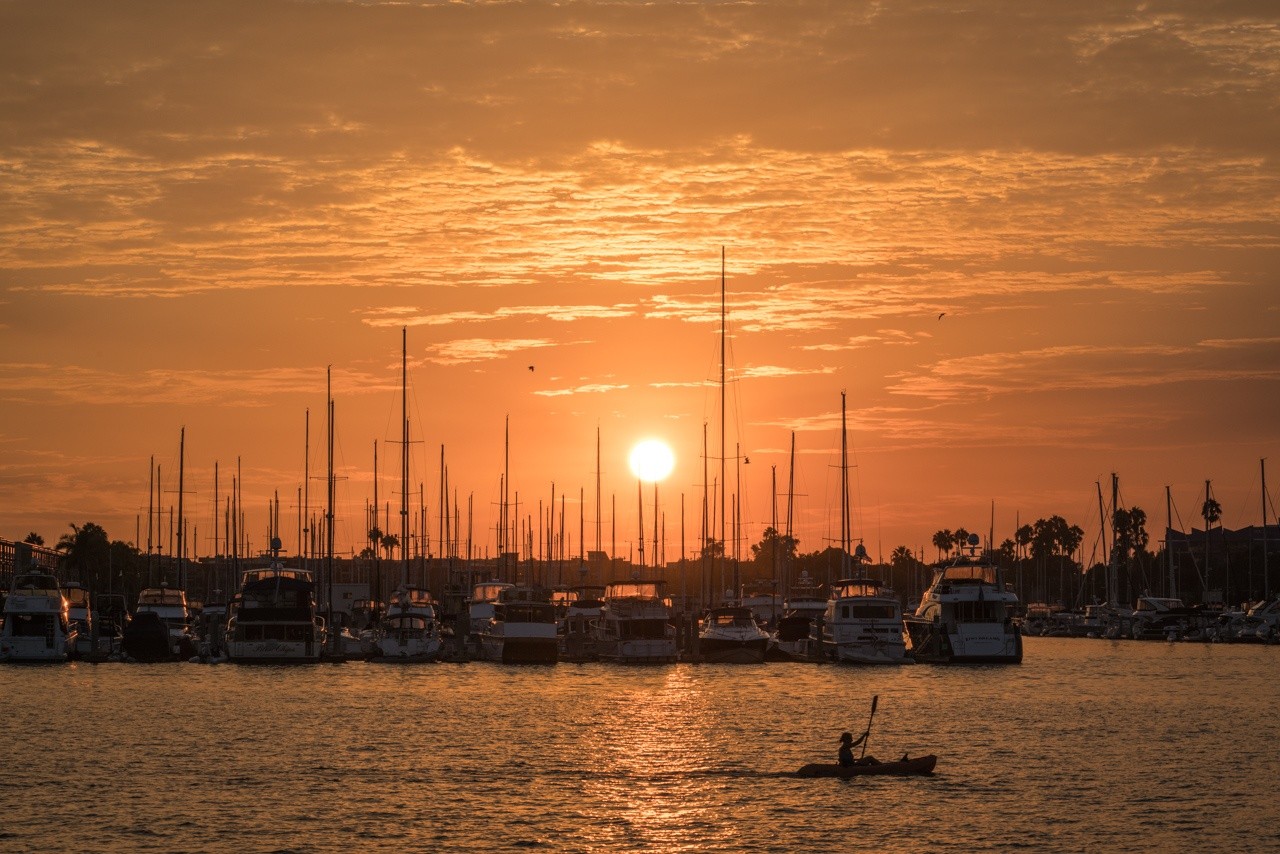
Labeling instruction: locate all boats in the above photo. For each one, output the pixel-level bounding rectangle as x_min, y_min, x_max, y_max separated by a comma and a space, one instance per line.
903, 536, 1030, 666
795, 752, 937, 778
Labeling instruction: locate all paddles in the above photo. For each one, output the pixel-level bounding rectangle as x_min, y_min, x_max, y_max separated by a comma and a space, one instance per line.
861, 695, 879, 760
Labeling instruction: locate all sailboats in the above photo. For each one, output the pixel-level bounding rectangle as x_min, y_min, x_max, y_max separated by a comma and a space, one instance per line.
0, 247, 1279, 671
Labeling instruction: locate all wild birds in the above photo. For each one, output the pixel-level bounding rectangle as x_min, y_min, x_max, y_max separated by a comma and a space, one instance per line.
528, 366, 534, 372
939, 312, 946, 320
744, 457, 751, 464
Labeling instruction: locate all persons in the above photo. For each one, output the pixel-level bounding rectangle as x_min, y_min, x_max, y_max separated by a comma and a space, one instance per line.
838, 732, 881, 767
